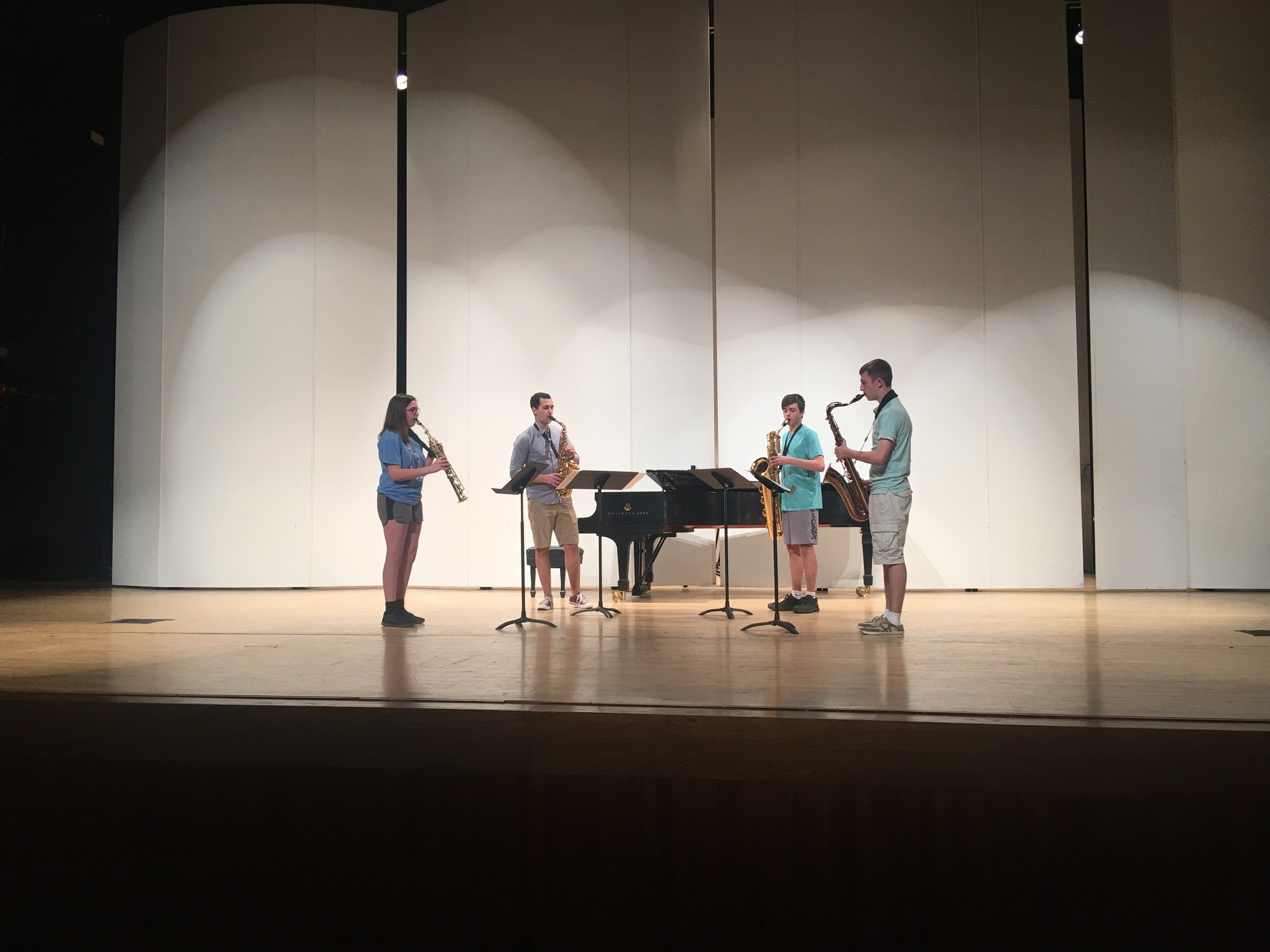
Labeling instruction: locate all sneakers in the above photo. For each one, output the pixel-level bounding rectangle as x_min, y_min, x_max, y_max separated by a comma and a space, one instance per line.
792, 595, 820, 613
398, 608, 425, 623
569, 592, 593, 608
860, 617, 904, 635
381, 609, 416, 627
537, 596, 554, 610
767, 593, 803, 611
857, 614, 884, 629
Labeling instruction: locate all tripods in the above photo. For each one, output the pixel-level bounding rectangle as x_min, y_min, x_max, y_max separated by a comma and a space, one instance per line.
740, 469, 799, 634
688, 468, 761, 620
491, 461, 558, 630
563, 469, 639, 618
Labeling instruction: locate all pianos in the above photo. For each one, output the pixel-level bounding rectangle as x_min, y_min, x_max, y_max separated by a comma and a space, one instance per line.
577, 465, 873, 604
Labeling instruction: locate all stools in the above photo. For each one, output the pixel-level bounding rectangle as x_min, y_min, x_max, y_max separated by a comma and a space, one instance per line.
526, 545, 584, 597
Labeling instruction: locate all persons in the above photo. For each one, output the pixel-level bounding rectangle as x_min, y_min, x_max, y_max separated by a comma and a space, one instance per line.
834, 358, 913, 635
509, 392, 593, 611
767, 394, 824, 614
377, 393, 451, 627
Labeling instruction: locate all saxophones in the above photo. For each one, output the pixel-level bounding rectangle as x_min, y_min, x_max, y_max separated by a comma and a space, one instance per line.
821, 392, 869, 522
548, 416, 580, 499
750, 418, 791, 541
415, 419, 469, 503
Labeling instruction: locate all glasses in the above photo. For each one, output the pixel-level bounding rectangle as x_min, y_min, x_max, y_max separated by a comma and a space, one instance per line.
405, 407, 420, 415
